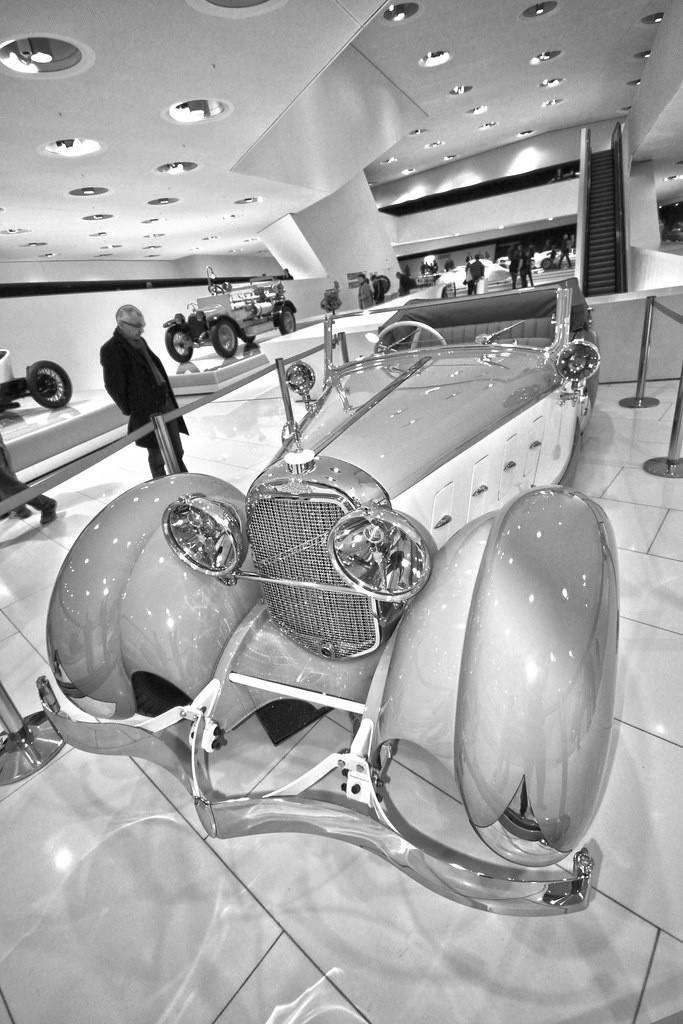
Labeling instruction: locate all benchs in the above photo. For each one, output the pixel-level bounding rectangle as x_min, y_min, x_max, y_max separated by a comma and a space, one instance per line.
393, 283, 556, 349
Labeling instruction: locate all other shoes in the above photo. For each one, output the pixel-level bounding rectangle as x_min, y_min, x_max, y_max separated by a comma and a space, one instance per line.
41, 500, 56, 524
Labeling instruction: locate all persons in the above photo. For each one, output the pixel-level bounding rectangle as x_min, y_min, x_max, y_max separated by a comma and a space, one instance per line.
421, 260, 438, 274
465, 251, 485, 295
99, 304, 190, 476
445, 258, 455, 272
358, 274, 372, 310
371, 275, 385, 304
396, 273, 410, 296
544, 235, 571, 270
0, 435, 57, 522
284, 269, 293, 279
509, 245, 533, 289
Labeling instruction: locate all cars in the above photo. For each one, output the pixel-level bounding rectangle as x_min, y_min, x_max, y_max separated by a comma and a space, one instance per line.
37, 278, 620, 914
163, 279, 297, 363
495, 250, 560, 269
0, 348, 73, 415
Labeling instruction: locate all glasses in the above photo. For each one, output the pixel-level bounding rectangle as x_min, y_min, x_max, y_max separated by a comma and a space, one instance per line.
120, 320, 145, 330
